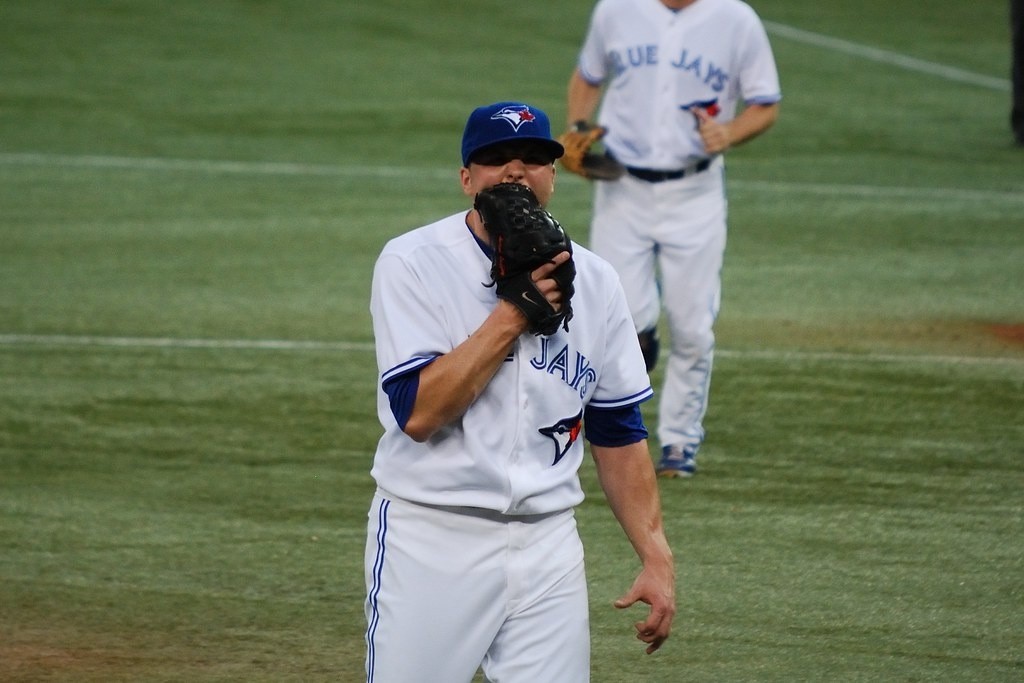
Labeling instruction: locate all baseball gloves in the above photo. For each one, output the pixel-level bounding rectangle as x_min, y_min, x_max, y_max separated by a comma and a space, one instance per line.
556, 122, 628, 182
472, 182, 575, 334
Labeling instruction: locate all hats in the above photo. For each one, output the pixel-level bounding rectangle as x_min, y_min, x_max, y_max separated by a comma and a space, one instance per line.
461, 102, 564, 167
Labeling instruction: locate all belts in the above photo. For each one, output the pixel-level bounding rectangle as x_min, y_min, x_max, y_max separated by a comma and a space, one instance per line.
605, 148, 716, 183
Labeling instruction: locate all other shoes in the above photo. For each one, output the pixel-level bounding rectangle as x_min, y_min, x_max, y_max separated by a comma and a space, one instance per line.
637, 325, 661, 372
656, 437, 701, 480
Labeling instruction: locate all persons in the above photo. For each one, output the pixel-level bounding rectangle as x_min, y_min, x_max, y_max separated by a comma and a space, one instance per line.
363, 99, 676, 683
563, 0, 784, 481
